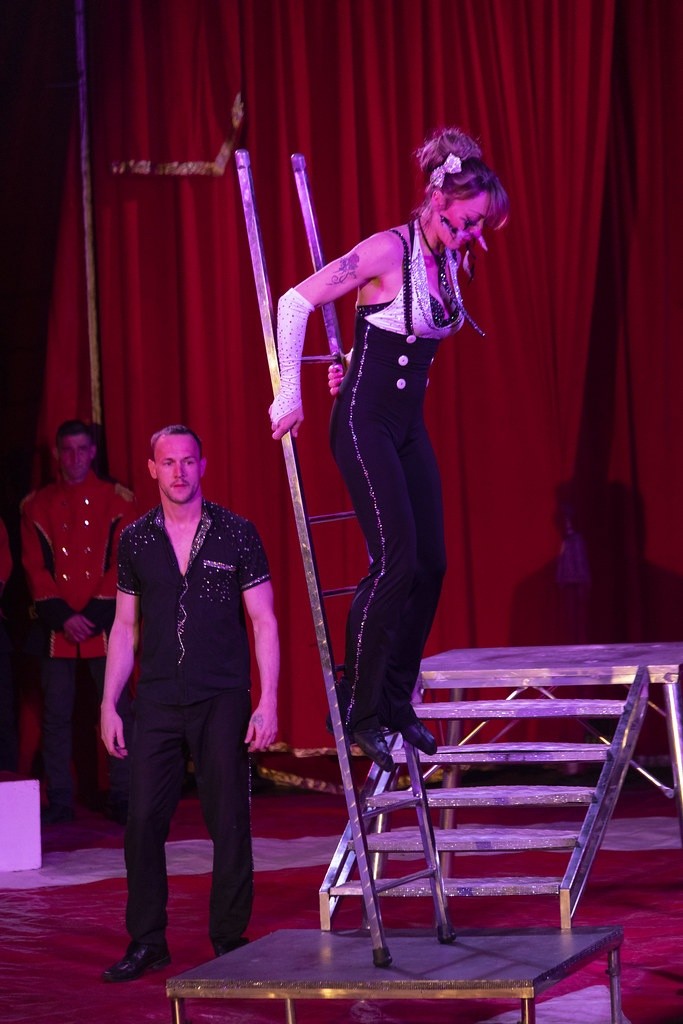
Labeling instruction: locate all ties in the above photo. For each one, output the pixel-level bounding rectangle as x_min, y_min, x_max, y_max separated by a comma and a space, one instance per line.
416, 216, 487, 337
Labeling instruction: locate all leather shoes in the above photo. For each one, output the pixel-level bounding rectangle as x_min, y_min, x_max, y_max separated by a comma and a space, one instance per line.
105, 946, 173, 981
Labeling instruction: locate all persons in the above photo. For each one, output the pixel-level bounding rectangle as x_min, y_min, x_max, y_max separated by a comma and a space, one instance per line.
268, 128, 508, 772
18, 419, 138, 824
100, 424, 280, 983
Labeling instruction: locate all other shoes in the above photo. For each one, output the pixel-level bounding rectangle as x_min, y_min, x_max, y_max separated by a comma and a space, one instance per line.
110, 802, 129, 823
39, 792, 72, 823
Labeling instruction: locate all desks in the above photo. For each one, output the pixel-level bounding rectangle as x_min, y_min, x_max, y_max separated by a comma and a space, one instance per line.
166, 925, 624, 1024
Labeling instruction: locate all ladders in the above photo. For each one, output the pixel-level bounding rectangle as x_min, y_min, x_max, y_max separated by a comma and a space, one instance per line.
231, 143, 459, 968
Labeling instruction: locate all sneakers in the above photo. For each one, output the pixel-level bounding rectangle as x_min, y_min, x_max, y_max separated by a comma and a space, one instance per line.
326, 710, 441, 771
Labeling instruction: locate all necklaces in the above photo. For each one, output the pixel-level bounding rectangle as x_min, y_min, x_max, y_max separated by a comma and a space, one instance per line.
419, 219, 487, 338
